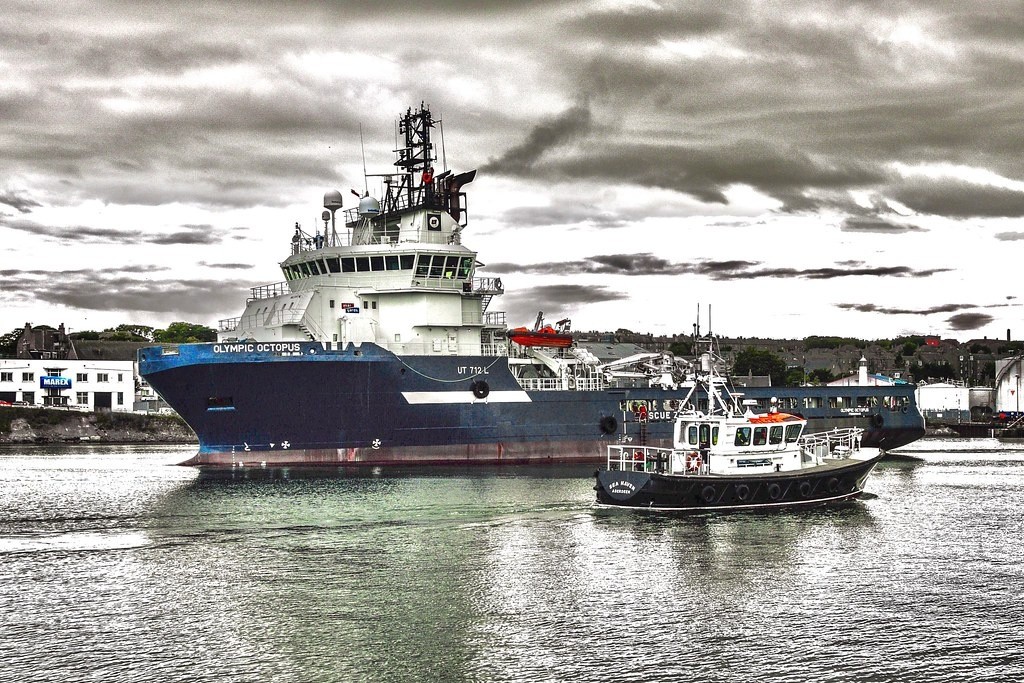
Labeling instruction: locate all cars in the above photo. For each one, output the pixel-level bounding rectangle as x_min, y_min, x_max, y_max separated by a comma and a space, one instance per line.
148, 409, 155, 414
157, 408, 162, 414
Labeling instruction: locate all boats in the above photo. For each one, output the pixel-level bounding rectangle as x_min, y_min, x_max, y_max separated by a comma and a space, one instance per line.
506, 325, 572, 348
594, 303, 886, 513
998, 415, 1024, 443
138, 101, 927, 475
946, 420, 1009, 438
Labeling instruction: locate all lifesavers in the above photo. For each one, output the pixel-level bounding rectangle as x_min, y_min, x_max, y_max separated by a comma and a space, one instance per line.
701, 485, 715, 503
798, 481, 811, 497
473, 380, 489, 399
736, 484, 749, 501
600, 416, 617, 434
687, 452, 701, 471
767, 483, 781, 500
826, 477, 838, 493
873, 413, 884, 428
